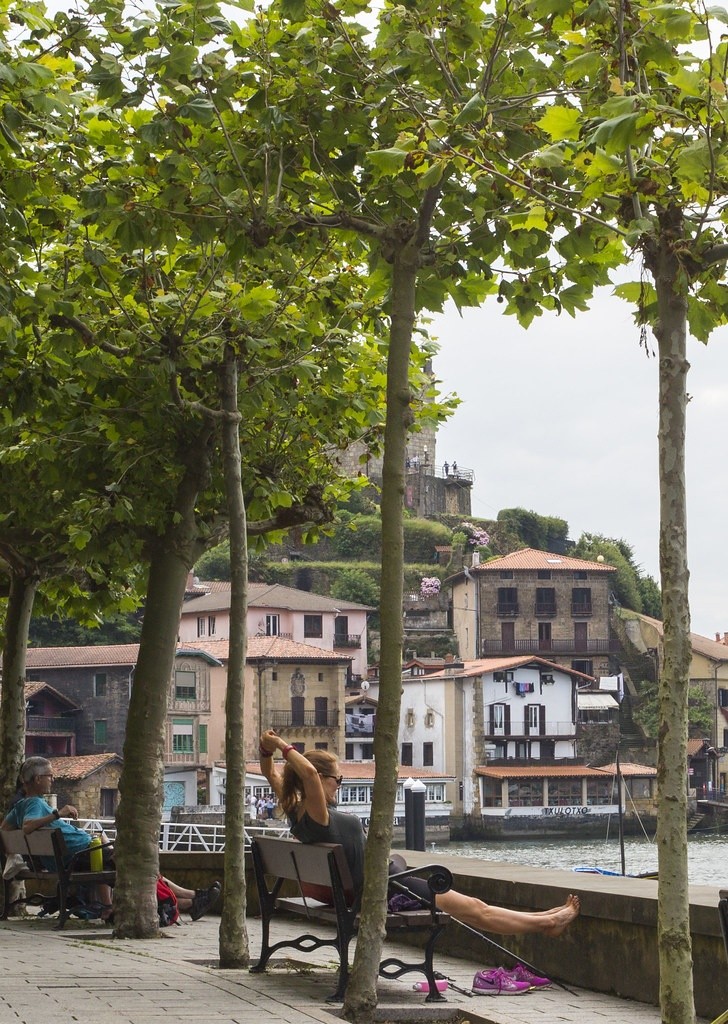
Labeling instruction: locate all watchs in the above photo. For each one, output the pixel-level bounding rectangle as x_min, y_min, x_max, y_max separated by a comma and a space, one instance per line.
52, 809, 60, 819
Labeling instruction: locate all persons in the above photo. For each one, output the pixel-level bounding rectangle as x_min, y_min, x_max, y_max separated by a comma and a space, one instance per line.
258, 729, 580, 938
244, 793, 275, 820
0, 756, 221, 921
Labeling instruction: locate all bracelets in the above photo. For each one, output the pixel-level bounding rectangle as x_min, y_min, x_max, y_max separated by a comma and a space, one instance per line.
282, 745, 297, 760
259, 744, 273, 758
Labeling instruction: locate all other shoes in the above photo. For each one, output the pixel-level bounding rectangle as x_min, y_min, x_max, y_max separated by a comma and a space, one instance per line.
69, 897, 103, 918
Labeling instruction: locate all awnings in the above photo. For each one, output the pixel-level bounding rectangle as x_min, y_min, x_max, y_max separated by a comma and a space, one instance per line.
577, 693, 619, 711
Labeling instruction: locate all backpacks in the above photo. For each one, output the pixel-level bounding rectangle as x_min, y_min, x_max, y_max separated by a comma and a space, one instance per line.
156, 879, 179, 926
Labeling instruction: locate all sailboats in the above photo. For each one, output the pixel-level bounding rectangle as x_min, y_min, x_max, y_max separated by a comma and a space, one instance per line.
575, 748, 660, 880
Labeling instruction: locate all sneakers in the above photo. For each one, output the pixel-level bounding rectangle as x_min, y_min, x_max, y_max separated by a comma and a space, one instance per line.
188, 887, 220, 921
473, 967, 532, 996
498, 963, 552, 991
194, 882, 221, 898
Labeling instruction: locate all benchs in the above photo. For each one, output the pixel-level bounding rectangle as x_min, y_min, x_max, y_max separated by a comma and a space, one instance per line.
710, 890, 728, 1024
249, 835, 454, 1003
0, 826, 116, 930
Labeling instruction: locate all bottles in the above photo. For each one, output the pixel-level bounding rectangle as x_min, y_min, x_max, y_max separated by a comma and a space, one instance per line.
90, 834, 103, 872
413, 980, 448, 991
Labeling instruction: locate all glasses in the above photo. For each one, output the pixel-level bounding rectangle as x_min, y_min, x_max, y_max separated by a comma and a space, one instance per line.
33, 774, 54, 780
323, 774, 344, 786
